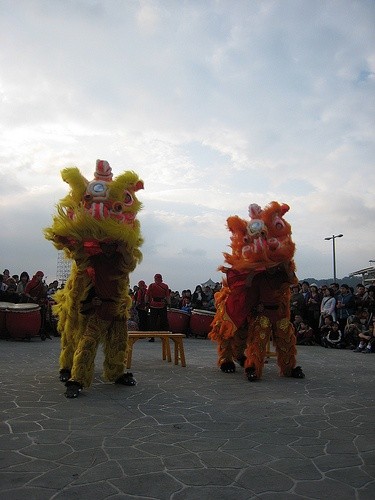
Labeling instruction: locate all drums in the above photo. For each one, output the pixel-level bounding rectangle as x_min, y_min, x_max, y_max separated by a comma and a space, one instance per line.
6, 303, 41, 339
189, 309, 216, 336
167, 308, 191, 332
0, 302, 15, 338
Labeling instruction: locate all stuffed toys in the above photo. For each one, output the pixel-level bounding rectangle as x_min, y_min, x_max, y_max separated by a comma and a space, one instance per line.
208, 200, 305, 382
47, 158, 145, 399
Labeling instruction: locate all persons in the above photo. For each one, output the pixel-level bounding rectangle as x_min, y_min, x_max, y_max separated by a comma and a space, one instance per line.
0, 267, 375, 354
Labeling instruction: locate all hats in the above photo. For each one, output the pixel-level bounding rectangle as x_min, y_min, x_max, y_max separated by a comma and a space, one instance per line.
310, 283, 317, 287
292, 285, 300, 291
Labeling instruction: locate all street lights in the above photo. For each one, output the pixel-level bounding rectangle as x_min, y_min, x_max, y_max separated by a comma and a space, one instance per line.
323, 233, 344, 283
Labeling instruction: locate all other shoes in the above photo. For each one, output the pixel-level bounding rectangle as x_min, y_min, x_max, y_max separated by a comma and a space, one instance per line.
59, 369, 71, 381
291, 366, 305, 378
234, 355, 247, 367
353, 347, 365, 351
245, 367, 257, 381
149, 339, 154, 342
362, 348, 374, 352
221, 362, 236, 373
64, 381, 81, 397
116, 373, 136, 385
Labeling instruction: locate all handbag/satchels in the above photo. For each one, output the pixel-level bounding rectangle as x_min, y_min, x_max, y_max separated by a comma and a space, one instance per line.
318, 315, 323, 328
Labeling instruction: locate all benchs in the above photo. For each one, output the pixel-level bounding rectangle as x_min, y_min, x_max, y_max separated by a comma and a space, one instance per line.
127, 330, 187, 369
